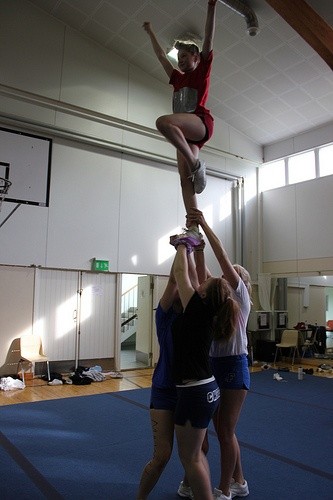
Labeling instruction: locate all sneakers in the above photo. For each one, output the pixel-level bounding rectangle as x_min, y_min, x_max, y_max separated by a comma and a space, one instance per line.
213, 488, 231, 500
231, 478, 249, 498
177, 481, 192, 497
188, 226, 199, 234
187, 159, 206, 194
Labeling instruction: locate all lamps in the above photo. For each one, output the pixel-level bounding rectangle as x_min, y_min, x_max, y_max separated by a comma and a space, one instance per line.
167, 33, 203, 62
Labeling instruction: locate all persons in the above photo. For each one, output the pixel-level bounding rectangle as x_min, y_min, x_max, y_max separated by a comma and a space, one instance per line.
140, 1, 217, 245
171, 237, 242, 500
136, 231, 211, 500
183, 206, 254, 500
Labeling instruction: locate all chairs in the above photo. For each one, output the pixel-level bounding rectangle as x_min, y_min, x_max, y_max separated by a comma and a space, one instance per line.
16, 336, 51, 384
274, 328, 319, 365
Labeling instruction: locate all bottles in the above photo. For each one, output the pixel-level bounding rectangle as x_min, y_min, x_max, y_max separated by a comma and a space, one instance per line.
298, 366, 303, 380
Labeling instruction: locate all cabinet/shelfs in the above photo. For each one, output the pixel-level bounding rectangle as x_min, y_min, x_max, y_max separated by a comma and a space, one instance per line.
256, 310, 287, 330
